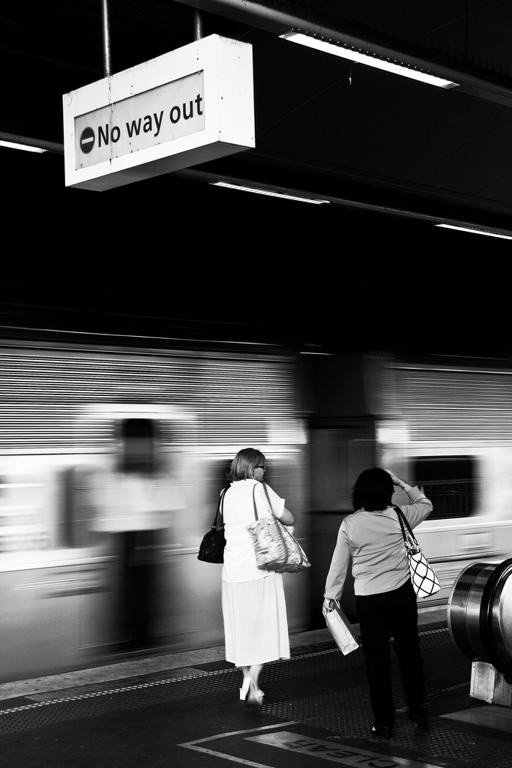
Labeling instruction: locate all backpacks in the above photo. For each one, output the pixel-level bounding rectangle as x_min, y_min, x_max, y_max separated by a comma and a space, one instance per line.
393, 540, 441, 602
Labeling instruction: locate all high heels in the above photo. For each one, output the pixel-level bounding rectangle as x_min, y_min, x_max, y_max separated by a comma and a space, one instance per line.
239, 673, 265, 705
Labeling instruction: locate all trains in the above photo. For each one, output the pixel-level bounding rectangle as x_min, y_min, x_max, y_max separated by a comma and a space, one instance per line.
0, 318, 512, 691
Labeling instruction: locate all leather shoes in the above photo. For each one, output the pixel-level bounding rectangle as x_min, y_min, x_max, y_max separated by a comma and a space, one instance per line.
412, 721, 430, 732
369, 723, 393, 739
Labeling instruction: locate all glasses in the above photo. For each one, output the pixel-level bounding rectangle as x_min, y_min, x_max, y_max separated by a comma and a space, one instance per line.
253, 464, 267, 469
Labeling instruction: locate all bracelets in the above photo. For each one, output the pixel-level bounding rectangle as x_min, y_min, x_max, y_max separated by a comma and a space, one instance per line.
400, 481, 408, 490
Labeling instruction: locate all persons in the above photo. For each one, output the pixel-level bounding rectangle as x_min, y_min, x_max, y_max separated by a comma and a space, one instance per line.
321, 466, 435, 742
216, 447, 296, 709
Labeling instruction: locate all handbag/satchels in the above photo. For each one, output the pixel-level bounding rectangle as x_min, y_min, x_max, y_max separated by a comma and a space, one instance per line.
195, 526, 227, 564
246, 517, 313, 574
321, 594, 360, 657
